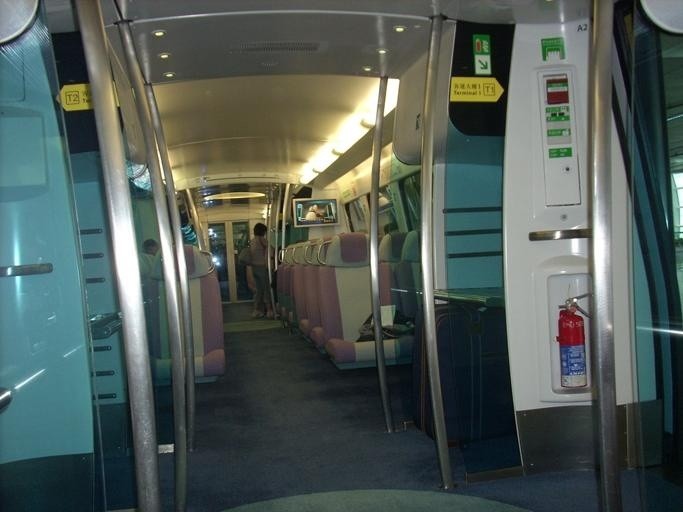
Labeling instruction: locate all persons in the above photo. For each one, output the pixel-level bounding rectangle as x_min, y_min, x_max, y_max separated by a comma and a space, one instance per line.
250, 223, 274, 318
305, 203, 324, 221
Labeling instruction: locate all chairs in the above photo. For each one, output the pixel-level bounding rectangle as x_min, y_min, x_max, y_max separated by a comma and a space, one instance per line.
141, 244, 227, 385
272, 229, 419, 369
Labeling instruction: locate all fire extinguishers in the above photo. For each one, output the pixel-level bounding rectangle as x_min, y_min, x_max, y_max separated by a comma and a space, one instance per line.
559, 293, 591, 388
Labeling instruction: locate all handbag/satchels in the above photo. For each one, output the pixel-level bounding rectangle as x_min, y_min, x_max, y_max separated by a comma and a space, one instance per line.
238, 247, 253, 266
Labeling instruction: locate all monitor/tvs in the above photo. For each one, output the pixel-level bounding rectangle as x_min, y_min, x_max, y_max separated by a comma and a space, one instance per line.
292, 196, 340, 228
